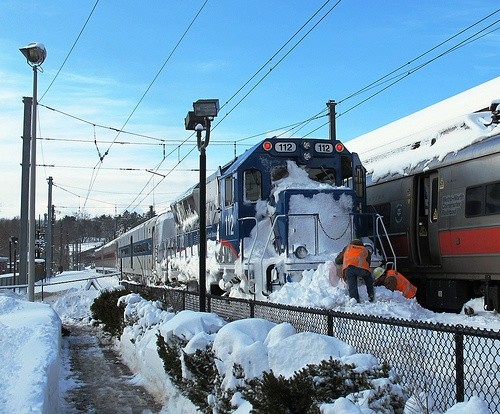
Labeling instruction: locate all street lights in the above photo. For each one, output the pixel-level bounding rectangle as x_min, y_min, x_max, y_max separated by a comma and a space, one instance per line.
19, 41, 46, 302
184, 99, 220, 312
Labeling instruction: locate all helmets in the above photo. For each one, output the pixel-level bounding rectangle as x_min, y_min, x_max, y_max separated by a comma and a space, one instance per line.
354, 239, 361, 241
373, 267, 385, 280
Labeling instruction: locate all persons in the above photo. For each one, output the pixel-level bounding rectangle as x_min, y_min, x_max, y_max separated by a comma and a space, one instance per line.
335, 239, 374, 303
372, 267, 417, 299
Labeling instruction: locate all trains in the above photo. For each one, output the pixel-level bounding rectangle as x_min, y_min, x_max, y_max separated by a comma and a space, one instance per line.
92, 136, 397, 300
340, 74, 500, 297
